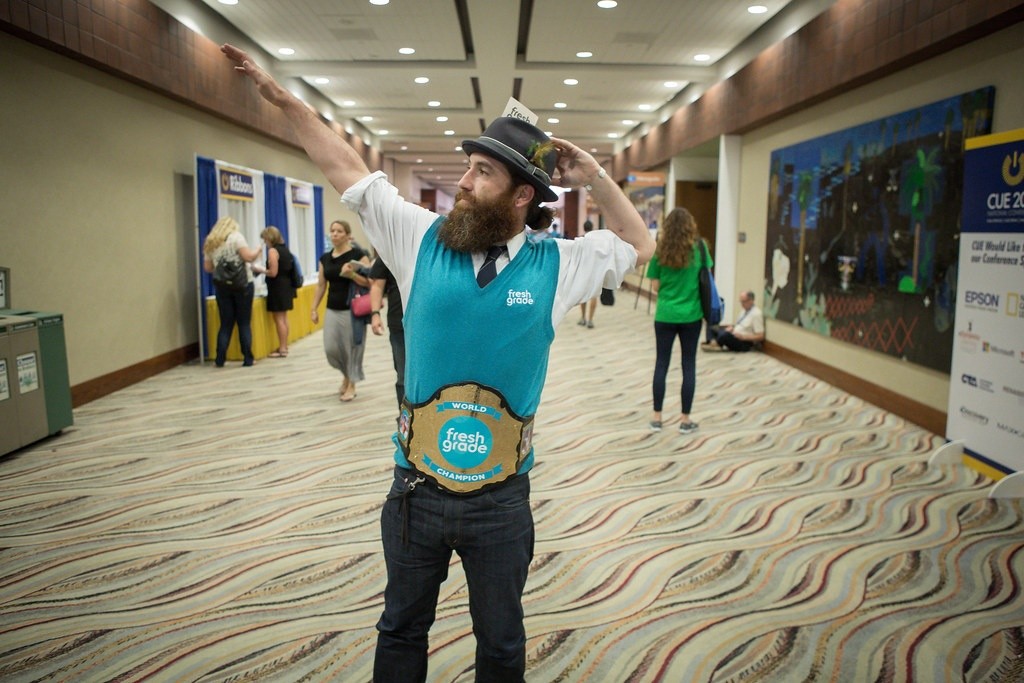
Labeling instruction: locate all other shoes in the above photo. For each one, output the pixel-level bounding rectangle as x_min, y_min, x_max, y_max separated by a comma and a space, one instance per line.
650, 421, 662, 431
589, 321, 594, 327
680, 422, 698, 434
701, 342, 721, 352
339, 380, 357, 402
578, 319, 587, 325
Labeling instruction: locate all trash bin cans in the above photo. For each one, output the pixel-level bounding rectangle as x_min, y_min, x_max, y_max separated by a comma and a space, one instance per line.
0, 309, 74, 458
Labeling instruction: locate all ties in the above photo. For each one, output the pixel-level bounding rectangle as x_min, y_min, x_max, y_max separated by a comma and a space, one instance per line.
477, 244, 509, 288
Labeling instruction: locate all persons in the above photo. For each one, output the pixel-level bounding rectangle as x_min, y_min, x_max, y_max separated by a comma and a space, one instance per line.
249, 225, 299, 356
645, 206, 712, 434
202, 215, 264, 367
549, 224, 562, 238
220, 41, 660, 683
310, 219, 375, 401
368, 238, 460, 437
702, 290, 765, 353
571, 220, 603, 329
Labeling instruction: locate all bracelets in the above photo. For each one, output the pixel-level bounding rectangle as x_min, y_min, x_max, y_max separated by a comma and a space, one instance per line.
370, 310, 380, 318
584, 164, 608, 196
311, 308, 317, 314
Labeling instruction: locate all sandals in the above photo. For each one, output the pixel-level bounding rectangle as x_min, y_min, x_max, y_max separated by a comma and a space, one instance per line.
267, 348, 288, 357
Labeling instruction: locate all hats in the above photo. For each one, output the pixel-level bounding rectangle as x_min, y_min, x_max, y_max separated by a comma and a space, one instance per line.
461, 117, 562, 202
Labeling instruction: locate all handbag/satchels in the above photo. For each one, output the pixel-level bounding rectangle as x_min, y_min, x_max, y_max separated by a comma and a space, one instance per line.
292, 255, 304, 288
601, 287, 615, 307
216, 257, 249, 292
697, 239, 726, 327
351, 293, 385, 316
